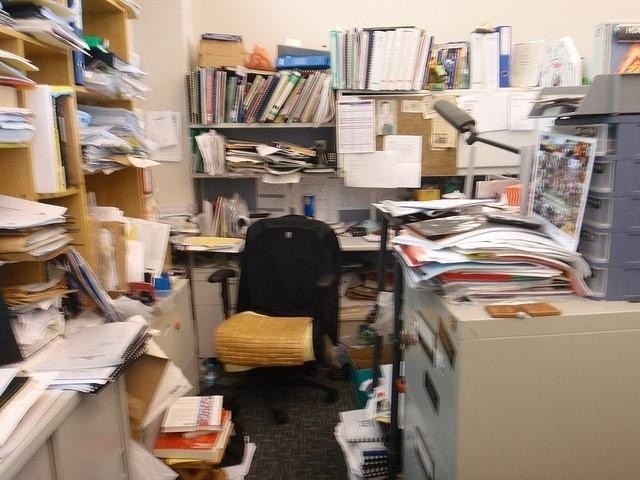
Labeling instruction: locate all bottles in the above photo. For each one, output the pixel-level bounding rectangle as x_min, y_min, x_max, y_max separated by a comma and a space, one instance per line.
458, 56, 469, 89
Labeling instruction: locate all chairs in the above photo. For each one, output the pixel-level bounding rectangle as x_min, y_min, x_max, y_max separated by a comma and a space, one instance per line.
199, 213, 346, 425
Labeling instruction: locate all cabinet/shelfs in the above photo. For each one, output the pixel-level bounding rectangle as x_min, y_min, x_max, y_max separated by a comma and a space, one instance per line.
402, 284, 639, 477
527, 73, 638, 305
180, 68, 405, 365
587, 21, 639, 87
1, 3, 201, 479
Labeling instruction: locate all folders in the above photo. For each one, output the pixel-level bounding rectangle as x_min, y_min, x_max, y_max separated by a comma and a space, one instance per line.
68, 0, 87, 85
495, 26, 510, 88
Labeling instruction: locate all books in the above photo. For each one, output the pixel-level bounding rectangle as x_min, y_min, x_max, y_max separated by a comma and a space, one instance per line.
1, 0, 174, 461
153, 396, 257, 479
175, 27, 607, 303
336, 332, 405, 478
